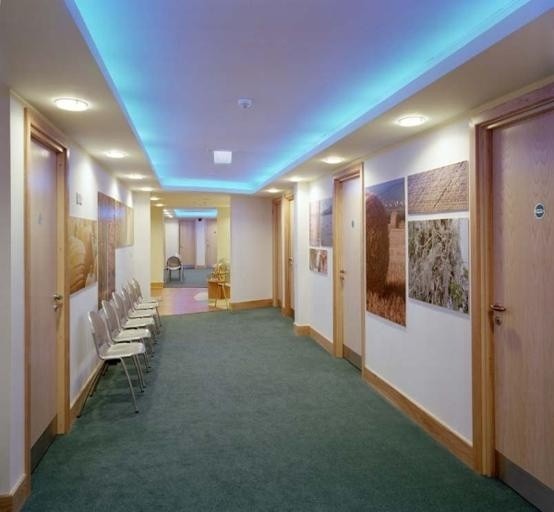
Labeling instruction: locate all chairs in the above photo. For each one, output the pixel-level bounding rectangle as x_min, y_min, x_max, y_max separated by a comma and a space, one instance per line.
166, 256, 183, 282
76, 277, 163, 422
